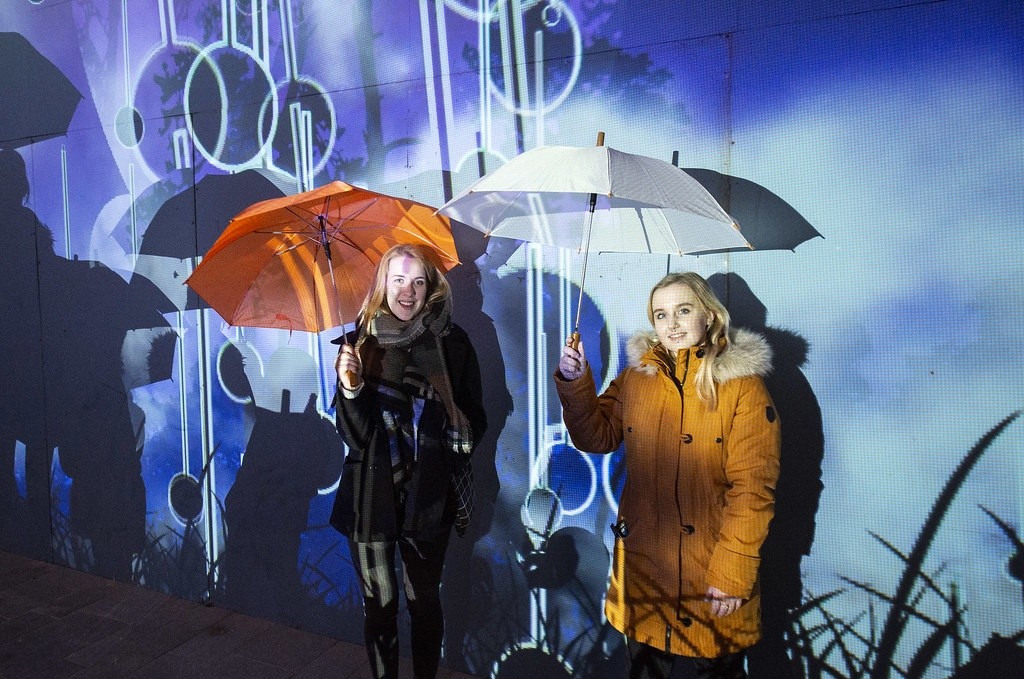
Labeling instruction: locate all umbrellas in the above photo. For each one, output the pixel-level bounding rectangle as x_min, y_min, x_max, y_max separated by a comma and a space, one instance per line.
181, 177, 464, 387
431, 131, 756, 360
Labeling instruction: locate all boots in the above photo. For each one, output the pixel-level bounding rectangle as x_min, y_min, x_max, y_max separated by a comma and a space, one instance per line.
361, 595, 400, 679
409, 597, 444, 678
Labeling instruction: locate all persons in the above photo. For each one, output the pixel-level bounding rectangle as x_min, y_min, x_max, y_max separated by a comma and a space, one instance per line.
328, 245, 490, 679
554, 271, 782, 679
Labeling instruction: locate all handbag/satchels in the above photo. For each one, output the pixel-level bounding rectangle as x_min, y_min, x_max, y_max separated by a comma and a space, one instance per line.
448, 457, 477, 539
